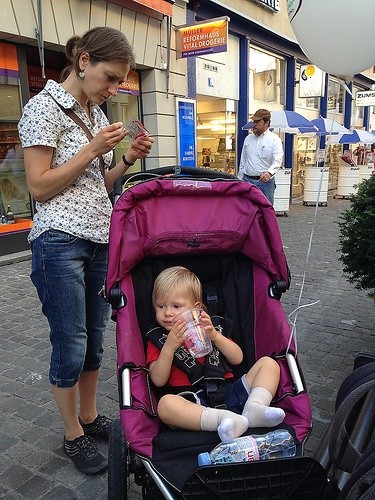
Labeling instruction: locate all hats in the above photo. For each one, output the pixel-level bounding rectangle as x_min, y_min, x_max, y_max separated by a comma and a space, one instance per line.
251, 109, 271, 120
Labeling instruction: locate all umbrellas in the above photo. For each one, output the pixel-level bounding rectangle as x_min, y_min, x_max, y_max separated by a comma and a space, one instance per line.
242, 110, 319, 138
326, 128, 375, 160
297, 117, 354, 167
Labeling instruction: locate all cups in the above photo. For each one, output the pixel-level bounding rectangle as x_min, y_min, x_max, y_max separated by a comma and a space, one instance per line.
172, 309, 213, 358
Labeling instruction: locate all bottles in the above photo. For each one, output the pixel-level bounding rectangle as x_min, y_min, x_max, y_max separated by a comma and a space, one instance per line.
198, 429, 296, 466
6, 205, 14, 224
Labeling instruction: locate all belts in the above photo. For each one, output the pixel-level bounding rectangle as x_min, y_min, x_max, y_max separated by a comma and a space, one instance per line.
244, 174, 261, 180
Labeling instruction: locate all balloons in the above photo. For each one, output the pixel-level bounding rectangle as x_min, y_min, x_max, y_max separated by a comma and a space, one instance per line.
286, 0, 375, 79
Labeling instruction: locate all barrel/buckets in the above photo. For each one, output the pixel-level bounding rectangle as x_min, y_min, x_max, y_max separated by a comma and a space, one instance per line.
303, 167, 329, 207
333, 165, 372, 199
273, 168, 291, 217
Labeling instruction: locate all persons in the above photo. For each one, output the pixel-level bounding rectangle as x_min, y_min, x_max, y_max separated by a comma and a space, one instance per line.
18, 27, 155, 475
371, 143, 375, 173
144, 266, 286, 443
238, 109, 284, 207
0, 178, 25, 215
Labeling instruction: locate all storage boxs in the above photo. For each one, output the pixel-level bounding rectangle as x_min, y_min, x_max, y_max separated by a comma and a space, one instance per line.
0, 217, 32, 256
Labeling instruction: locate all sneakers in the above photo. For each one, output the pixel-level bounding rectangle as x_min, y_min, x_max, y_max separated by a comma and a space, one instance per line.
63, 432, 108, 476
76, 414, 114, 440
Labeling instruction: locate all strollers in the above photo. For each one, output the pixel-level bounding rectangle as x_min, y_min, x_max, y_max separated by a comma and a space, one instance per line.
108, 164, 328, 500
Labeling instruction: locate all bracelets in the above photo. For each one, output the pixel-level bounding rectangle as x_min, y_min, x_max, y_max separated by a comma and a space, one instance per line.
121, 154, 136, 167
